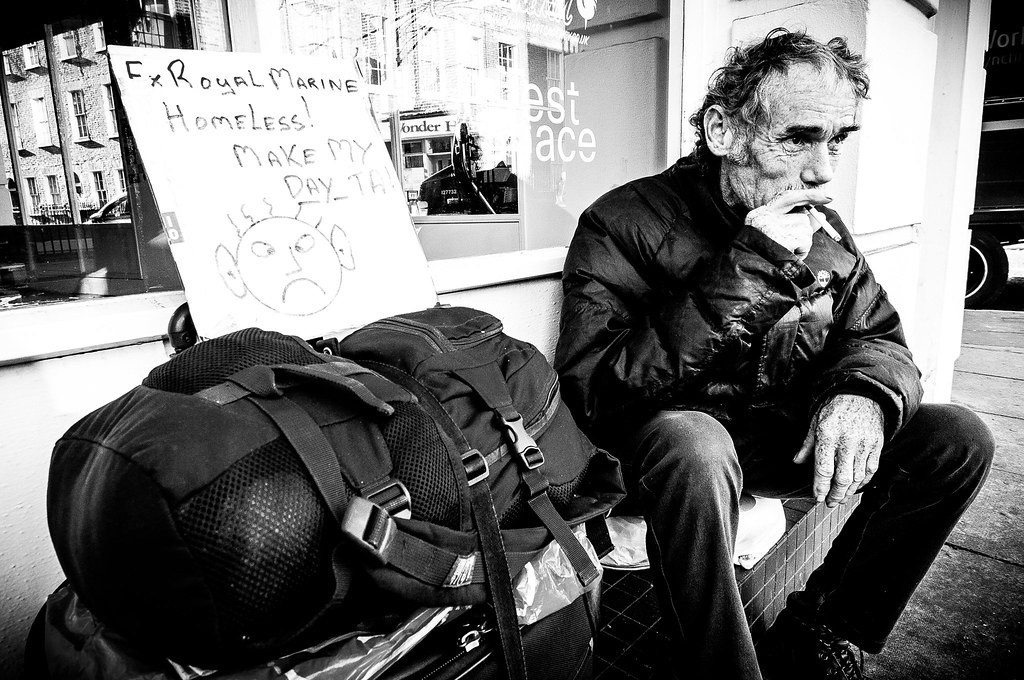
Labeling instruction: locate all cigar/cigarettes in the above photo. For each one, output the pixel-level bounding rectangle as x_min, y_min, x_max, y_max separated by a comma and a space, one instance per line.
805, 204, 842, 242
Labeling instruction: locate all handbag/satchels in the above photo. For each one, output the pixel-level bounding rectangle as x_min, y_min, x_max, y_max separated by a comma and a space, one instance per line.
315, 304, 623, 588
47, 323, 476, 677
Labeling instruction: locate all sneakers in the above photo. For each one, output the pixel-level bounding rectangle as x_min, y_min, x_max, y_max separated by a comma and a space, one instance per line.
754, 588, 868, 680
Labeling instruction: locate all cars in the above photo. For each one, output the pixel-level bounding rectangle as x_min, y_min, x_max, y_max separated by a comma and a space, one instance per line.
85, 191, 132, 224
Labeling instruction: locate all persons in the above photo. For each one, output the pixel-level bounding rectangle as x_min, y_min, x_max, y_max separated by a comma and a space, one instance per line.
556, 25, 995, 680
